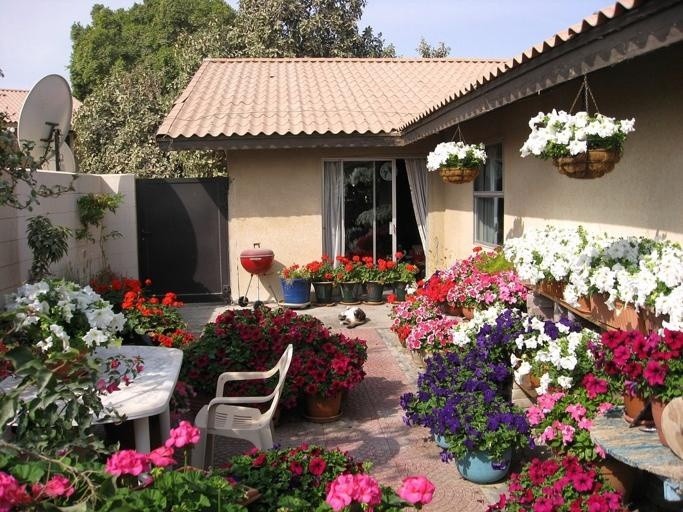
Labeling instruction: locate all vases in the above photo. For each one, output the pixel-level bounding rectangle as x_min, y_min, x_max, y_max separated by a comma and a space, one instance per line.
440, 166, 478, 183
550, 147, 621, 179
280, 279, 406, 308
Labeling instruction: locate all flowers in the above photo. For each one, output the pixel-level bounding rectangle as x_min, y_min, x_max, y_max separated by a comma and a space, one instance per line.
173, 305, 368, 421
517, 108, 636, 162
0, 269, 191, 353
426, 142, 489, 172
278, 251, 417, 287
0, 415, 436, 512
389, 220, 683, 512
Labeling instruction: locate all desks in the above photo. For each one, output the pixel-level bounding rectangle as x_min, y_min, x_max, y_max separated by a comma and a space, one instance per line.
1, 345, 185, 474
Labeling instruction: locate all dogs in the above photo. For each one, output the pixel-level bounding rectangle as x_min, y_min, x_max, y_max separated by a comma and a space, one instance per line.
337, 305, 367, 329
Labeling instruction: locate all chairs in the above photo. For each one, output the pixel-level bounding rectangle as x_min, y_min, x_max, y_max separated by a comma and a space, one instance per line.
192, 343, 293, 472
